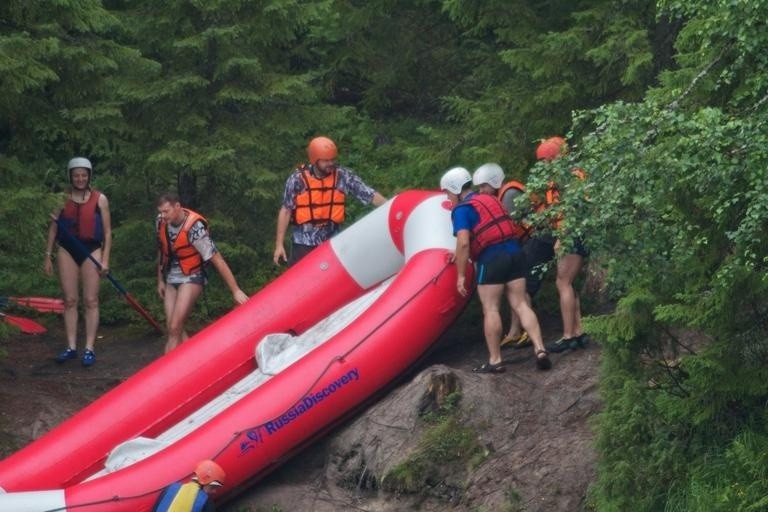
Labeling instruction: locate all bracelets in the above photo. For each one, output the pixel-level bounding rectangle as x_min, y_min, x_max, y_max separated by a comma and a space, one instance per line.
44, 251, 52, 257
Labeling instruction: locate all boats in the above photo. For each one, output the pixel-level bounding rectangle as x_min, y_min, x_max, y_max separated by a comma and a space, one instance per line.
0, 187, 476, 512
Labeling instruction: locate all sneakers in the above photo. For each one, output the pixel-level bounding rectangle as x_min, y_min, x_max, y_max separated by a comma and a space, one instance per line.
80, 348, 97, 366
55, 348, 79, 363
543, 332, 589, 353
500, 331, 532, 349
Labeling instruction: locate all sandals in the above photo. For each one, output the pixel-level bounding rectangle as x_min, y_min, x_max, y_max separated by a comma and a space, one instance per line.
470, 361, 506, 374
534, 350, 552, 369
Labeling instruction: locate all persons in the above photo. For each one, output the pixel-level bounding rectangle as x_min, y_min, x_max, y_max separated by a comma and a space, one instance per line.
537, 136, 593, 353
272, 135, 389, 273
43, 157, 112, 368
438, 166, 553, 375
472, 162, 555, 350
154, 188, 251, 355
151, 458, 226, 511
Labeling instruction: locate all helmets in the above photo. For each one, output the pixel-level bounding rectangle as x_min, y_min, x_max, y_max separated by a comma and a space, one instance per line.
307, 135, 339, 167
439, 167, 473, 196
190, 458, 226, 489
66, 157, 93, 187
472, 162, 507, 189
535, 135, 569, 164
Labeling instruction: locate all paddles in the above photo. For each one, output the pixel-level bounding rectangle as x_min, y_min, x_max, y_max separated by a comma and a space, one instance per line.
0, 312, 49, 334
0, 296, 65, 313
48, 212, 160, 333
